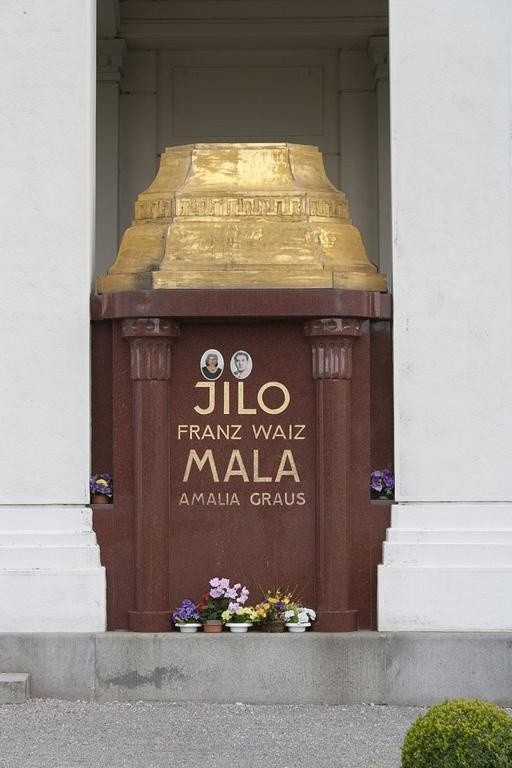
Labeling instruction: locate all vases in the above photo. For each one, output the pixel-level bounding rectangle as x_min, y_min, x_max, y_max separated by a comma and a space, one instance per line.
174, 620, 310, 633
90, 492, 112, 506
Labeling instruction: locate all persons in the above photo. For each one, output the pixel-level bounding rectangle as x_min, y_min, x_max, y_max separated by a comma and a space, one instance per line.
202, 353, 222, 379
232, 352, 252, 379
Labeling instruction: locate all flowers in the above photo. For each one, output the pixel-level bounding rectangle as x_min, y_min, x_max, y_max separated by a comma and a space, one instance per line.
368, 470, 394, 496
90, 474, 112, 496
160, 577, 311, 622
